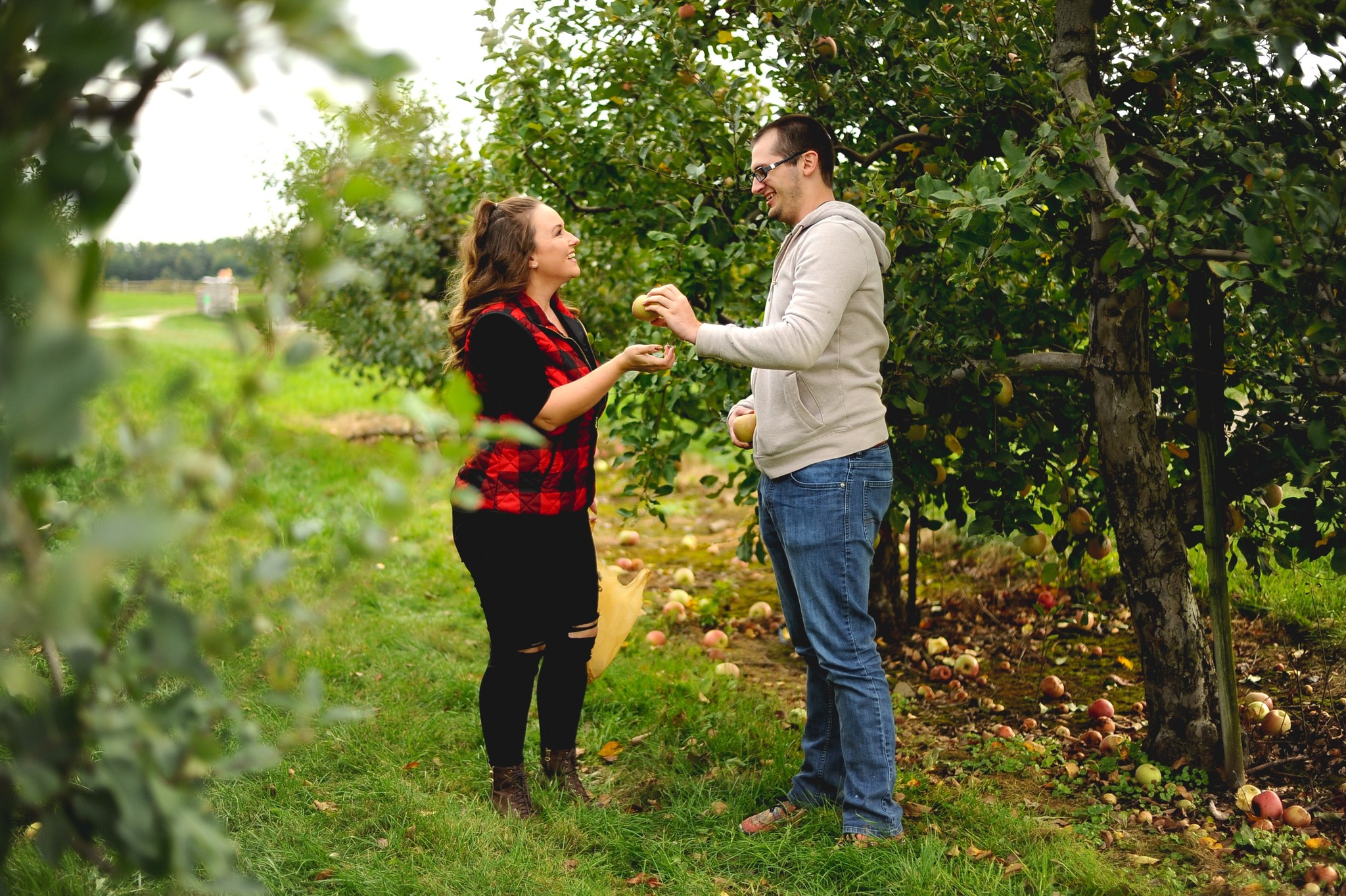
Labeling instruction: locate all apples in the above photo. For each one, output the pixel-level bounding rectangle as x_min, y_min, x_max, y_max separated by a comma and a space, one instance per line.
592, 0, 1346, 895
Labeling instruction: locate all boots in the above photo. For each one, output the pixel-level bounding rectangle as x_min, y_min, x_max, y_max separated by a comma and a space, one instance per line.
540, 747, 593, 807
488, 762, 539, 820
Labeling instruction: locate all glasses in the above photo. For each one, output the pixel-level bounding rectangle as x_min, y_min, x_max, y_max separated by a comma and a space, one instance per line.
748, 150, 808, 184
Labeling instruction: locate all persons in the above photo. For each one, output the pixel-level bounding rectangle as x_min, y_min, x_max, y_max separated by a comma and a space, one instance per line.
641, 114, 903, 851
439, 196, 675, 821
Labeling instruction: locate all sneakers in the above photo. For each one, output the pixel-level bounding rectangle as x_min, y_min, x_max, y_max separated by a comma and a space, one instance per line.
830, 831, 904, 852
739, 801, 801, 835
